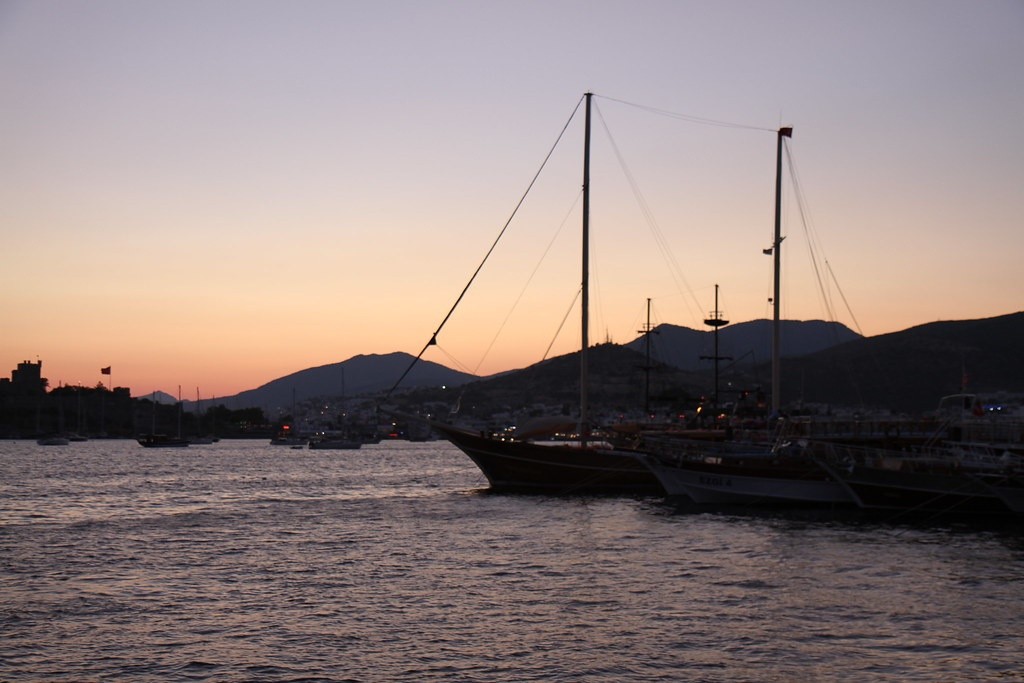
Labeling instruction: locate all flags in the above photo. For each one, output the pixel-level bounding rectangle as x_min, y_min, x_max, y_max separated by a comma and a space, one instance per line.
101, 366, 110, 375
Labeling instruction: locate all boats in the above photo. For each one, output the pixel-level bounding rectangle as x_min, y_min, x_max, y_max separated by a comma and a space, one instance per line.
135, 386, 221, 448
37, 430, 87, 446
434, 92, 1024, 528
270, 423, 381, 450
